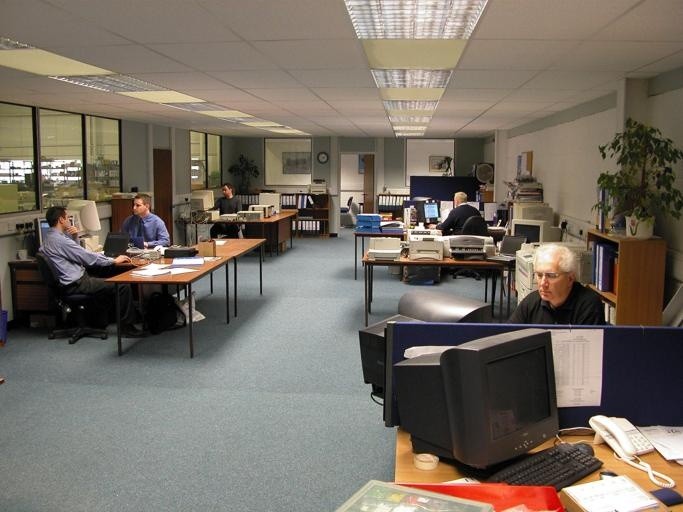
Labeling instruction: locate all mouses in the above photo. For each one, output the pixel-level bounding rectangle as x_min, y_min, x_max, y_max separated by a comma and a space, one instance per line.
573, 440, 599, 460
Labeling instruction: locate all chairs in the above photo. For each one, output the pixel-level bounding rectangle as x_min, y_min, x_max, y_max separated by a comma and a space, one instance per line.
340, 196, 353, 227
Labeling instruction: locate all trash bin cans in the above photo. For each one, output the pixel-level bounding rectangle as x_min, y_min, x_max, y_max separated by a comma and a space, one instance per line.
0, 310, 8, 347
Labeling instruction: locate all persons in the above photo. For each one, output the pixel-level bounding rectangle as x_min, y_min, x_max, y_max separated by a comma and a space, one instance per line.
207, 183, 243, 239
427, 190, 484, 235
505, 243, 607, 323
121, 193, 171, 250
42, 206, 143, 338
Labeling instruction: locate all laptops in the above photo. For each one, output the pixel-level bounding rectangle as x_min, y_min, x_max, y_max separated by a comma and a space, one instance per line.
424, 202, 443, 229
485, 235, 529, 265
103, 231, 144, 259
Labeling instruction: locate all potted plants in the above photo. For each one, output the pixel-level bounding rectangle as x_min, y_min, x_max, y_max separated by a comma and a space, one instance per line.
590, 117, 683, 240
228, 154, 263, 193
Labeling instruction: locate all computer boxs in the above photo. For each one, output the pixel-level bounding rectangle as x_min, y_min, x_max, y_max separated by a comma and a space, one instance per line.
237, 193, 282, 221
192, 210, 220, 221
78, 235, 100, 252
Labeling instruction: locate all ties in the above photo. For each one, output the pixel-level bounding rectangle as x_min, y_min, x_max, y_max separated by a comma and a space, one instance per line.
137, 219, 142, 237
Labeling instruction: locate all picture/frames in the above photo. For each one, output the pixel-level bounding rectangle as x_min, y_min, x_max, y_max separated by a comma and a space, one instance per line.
282, 152, 311, 174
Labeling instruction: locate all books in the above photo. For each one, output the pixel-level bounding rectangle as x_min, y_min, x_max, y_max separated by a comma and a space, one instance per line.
503, 175, 544, 205
559, 475, 661, 512
332, 480, 494, 512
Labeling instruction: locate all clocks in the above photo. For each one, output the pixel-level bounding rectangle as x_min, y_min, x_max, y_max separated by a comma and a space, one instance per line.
318, 152, 328, 164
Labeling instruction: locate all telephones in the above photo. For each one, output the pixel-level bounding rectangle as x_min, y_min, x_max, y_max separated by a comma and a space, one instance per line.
588, 414, 654, 461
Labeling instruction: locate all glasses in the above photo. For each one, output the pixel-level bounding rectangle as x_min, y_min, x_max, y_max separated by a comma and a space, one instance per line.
532, 271, 568, 280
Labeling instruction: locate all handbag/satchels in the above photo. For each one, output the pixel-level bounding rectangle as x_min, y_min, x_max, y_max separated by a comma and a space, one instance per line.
164, 248, 198, 258
142, 292, 187, 331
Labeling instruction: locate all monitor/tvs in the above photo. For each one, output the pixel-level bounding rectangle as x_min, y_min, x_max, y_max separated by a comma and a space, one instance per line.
359, 288, 494, 400
402, 200, 426, 223
187, 187, 216, 212
507, 218, 565, 253
55, 197, 102, 236
392, 326, 561, 469
31, 217, 77, 254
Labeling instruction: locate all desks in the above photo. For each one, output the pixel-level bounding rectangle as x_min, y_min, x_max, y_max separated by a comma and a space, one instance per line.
395, 429, 683, 512
175, 209, 299, 262
9, 238, 265, 359
353, 226, 515, 327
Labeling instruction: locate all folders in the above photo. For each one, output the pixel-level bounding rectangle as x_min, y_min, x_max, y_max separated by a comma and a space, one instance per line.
293, 220, 321, 238
235, 193, 259, 210
379, 195, 410, 212
279, 194, 316, 210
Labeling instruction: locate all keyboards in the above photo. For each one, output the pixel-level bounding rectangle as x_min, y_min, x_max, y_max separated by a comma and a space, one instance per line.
469, 443, 603, 493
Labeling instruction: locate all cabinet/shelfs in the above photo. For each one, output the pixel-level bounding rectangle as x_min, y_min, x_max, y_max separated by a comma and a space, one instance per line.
111, 198, 133, 232
586, 229, 668, 326
378, 194, 410, 220
235, 193, 330, 239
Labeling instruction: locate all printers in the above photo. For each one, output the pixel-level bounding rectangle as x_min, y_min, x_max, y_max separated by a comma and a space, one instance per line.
515, 242, 595, 307
367, 235, 496, 261
